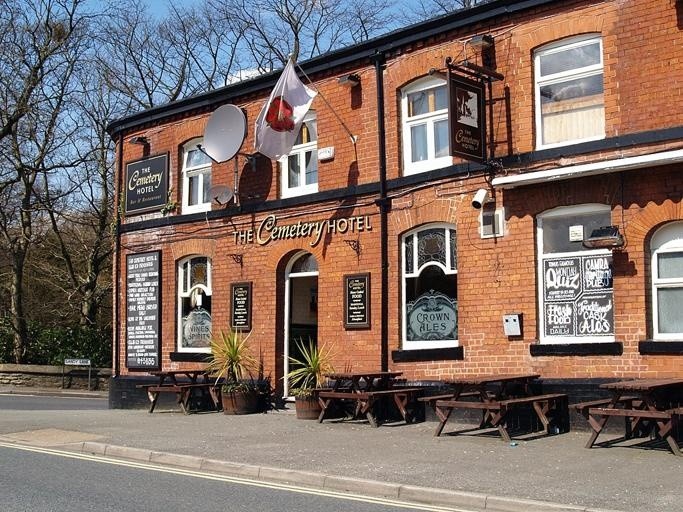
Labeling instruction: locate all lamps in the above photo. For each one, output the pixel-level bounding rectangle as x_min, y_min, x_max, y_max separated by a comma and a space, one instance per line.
470, 36, 494, 47
583, 227, 625, 252
337, 75, 360, 87
128, 137, 148, 145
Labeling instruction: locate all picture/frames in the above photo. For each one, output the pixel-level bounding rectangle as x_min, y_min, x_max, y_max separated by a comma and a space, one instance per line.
343, 272, 371, 330
229, 281, 252, 332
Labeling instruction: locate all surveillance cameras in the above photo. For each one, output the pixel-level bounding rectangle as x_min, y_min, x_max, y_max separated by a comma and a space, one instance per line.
471, 189, 489, 210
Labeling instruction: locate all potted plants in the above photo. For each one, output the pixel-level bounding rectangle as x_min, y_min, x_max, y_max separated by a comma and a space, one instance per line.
281, 338, 337, 420
206, 326, 263, 415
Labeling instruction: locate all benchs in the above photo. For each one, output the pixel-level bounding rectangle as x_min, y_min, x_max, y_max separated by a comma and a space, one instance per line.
571, 398, 683, 458
310, 385, 423, 429
137, 382, 221, 414
415, 390, 569, 445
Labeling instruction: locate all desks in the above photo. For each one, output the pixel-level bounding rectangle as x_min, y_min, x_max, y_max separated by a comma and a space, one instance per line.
147, 369, 219, 415
581, 379, 683, 455
440, 374, 553, 442
318, 372, 413, 425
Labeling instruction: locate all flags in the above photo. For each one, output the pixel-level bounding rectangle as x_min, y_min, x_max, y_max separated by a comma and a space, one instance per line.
254, 57, 317, 161
